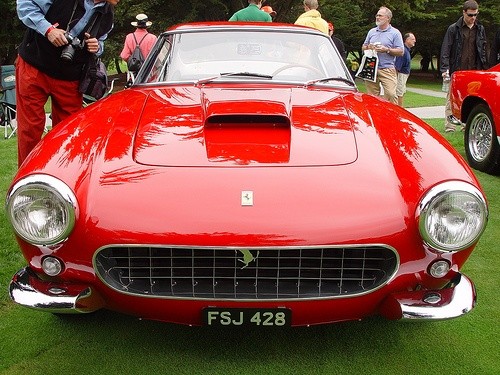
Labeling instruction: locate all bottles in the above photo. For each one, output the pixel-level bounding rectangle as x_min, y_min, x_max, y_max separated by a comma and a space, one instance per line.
442, 70, 450, 92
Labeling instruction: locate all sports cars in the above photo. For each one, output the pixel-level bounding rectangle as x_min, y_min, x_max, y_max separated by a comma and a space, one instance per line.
450, 61, 500, 172
6, 21, 490, 332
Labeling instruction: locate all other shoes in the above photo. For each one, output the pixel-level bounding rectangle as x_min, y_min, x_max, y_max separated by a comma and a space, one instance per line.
460, 126, 465, 132
445, 127, 456, 133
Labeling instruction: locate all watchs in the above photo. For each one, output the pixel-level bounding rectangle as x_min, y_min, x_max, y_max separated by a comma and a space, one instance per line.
386, 47, 390, 53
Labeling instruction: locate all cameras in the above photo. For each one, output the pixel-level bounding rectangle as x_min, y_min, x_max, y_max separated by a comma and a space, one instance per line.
59, 32, 81, 64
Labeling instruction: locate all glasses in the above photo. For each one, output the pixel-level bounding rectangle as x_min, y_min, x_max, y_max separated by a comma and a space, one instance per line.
465, 12, 479, 17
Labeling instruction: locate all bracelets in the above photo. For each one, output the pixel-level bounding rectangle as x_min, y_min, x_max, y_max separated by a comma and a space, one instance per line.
367, 45, 368, 49
46, 23, 59, 38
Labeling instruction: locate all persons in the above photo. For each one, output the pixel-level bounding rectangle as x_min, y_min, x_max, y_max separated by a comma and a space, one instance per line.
327, 22, 346, 62
394, 33, 416, 107
294, 0, 329, 35
120, 14, 163, 83
228, 0, 272, 22
261, 6, 277, 21
362, 6, 404, 104
16, 0, 120, 170
346, 43, 360, 81
440, 0, 490, 133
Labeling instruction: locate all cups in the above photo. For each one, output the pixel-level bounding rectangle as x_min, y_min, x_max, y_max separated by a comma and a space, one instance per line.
374, 42, 382, 50
